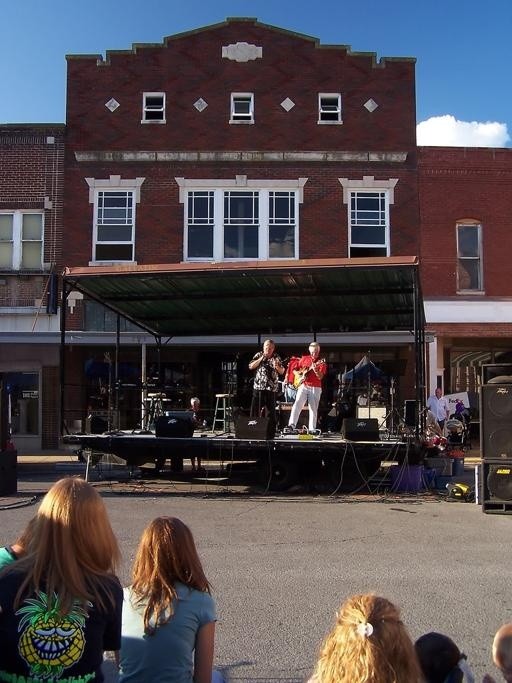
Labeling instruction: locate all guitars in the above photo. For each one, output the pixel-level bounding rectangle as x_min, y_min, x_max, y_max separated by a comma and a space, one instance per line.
293, 358, 325, 388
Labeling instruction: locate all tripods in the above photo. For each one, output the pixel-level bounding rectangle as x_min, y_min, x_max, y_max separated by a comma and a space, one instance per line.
378, 384, 409, 434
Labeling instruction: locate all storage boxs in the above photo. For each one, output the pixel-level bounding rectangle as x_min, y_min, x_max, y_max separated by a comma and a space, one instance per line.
391, 464, 424, 492
424, 456, 455, 477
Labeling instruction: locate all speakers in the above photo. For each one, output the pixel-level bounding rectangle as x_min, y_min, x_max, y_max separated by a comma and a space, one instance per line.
404, 399, 416, 427
156, 415, 195, 437
343, 418, 379, 440
479, 363, 512, 515
84, 415, 111, 434
235, 416, 276, 438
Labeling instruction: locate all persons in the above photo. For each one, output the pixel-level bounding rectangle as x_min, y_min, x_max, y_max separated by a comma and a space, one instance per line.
414, 630, 470, 683
304, 591, 427, 683
183, 395, 207, 473
0, 475, 124, 682
483, 623, 511, 682
100, 514, 227, 682
426, 387, 450, 430
248, 339, 284, 440
283, 341, 329, 435
0, 513, 34, 570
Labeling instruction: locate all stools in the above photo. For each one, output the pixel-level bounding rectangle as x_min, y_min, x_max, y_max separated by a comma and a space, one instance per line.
147, 393, 167, 431
212, 393, 232, 434
